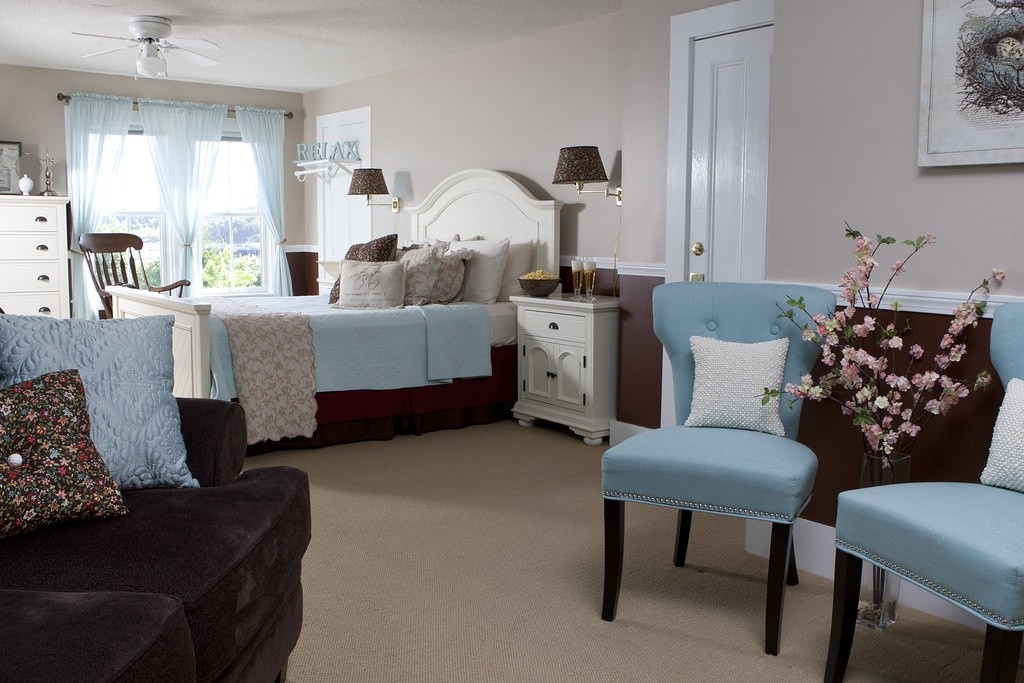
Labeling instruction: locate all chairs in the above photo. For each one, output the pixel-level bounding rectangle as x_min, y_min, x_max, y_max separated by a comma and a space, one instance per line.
601, 281, 838, 656
824, 300, 1024, 683
78, 232, 190, 319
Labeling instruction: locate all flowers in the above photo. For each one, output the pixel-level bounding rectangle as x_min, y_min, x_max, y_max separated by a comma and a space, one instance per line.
752, 219, 1007, 623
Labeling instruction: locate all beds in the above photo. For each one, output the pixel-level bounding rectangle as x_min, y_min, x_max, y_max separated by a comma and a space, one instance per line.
104, 168, 565, 458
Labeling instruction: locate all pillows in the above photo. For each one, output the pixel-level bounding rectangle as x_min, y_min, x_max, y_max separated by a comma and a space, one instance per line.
329, 234, 533, 310
0, 369, 129, 539
684, 336, 790, 437
979, 378, 1024, 494
0, 311, 203, 491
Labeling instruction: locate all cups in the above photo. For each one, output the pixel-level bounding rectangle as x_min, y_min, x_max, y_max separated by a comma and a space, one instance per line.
571, 260, 583, 300
583, 261, 596, 301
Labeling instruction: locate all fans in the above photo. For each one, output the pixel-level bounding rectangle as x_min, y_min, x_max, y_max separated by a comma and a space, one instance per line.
71, 16, 221, 68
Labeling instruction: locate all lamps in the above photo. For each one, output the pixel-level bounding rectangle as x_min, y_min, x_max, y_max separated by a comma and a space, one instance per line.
347, 168, 399, 213
135, 44, 168, 80
552, 145, 623, 206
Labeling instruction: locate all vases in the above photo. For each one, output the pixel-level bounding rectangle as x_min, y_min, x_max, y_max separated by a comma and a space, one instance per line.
854, 449, 911, 632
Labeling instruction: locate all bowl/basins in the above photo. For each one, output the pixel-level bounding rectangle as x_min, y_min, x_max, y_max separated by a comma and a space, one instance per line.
519, 279, 562, 297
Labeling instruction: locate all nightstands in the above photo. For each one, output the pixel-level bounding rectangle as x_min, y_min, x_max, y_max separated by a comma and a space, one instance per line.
508, 292, 620, 445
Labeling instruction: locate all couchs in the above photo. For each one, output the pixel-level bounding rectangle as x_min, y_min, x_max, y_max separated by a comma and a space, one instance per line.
0, 397, 312, 683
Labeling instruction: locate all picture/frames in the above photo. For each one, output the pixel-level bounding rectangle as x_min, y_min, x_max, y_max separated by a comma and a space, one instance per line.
916, 0, 1024, 167
0, 140, 23, 195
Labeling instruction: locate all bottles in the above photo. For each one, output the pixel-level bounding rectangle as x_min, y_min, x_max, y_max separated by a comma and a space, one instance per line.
18, 175, 33, 195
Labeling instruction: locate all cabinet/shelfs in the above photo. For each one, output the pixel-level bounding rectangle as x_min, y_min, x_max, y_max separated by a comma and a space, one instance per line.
0, 195, 70, 320
